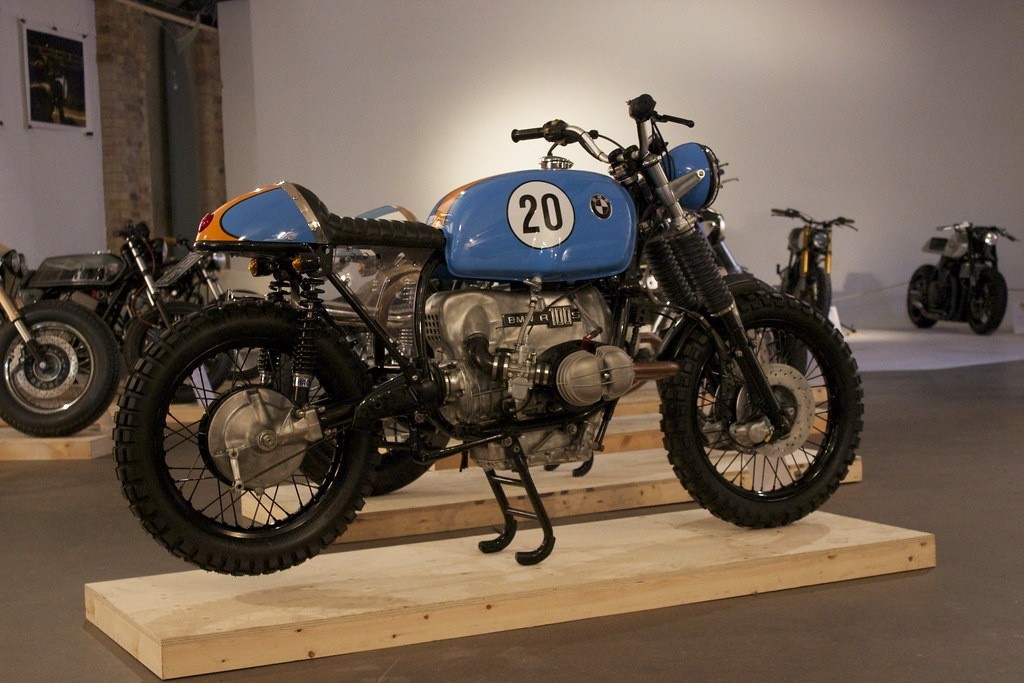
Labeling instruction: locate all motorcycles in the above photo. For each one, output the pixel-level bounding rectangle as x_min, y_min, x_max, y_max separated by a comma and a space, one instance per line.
770, 206, 859, 316
111, 92, 871, 576
14, 218, 235, 402
906, 222, 1020, 336
1, 242, 119, 437
